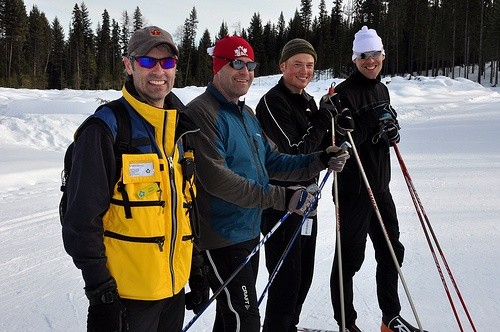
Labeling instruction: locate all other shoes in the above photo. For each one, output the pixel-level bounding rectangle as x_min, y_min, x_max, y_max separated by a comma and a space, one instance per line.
381, 315, 429, 332
339, 325, 361, 332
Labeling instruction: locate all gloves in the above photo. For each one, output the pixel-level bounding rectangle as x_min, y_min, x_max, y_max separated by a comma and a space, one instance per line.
320, 92, 346, 118
334, 108, 354, 135
373, 103, 397, 122
185, 254, 209, 314
372, 121, 400, 144
324, 146, 350, 172
85, 277, 131, 332
285, 185, 318, 217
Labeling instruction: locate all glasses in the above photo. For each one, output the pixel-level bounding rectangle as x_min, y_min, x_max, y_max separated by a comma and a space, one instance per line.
131, 56, 178, 69
212, 55, 258, 71
356, 51, 381, 60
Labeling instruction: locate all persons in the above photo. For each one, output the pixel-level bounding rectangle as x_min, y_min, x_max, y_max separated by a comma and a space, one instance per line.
254, 37, 328, 332
186, 36, 349, 332
59, 27, 210, 332
319, 25, 429, 332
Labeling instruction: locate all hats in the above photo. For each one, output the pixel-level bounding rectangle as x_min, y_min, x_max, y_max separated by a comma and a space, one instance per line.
213, 36, 254, 73
352, 26, 386, 61
127, 26, 180, 61
279, 38, 318, 65
